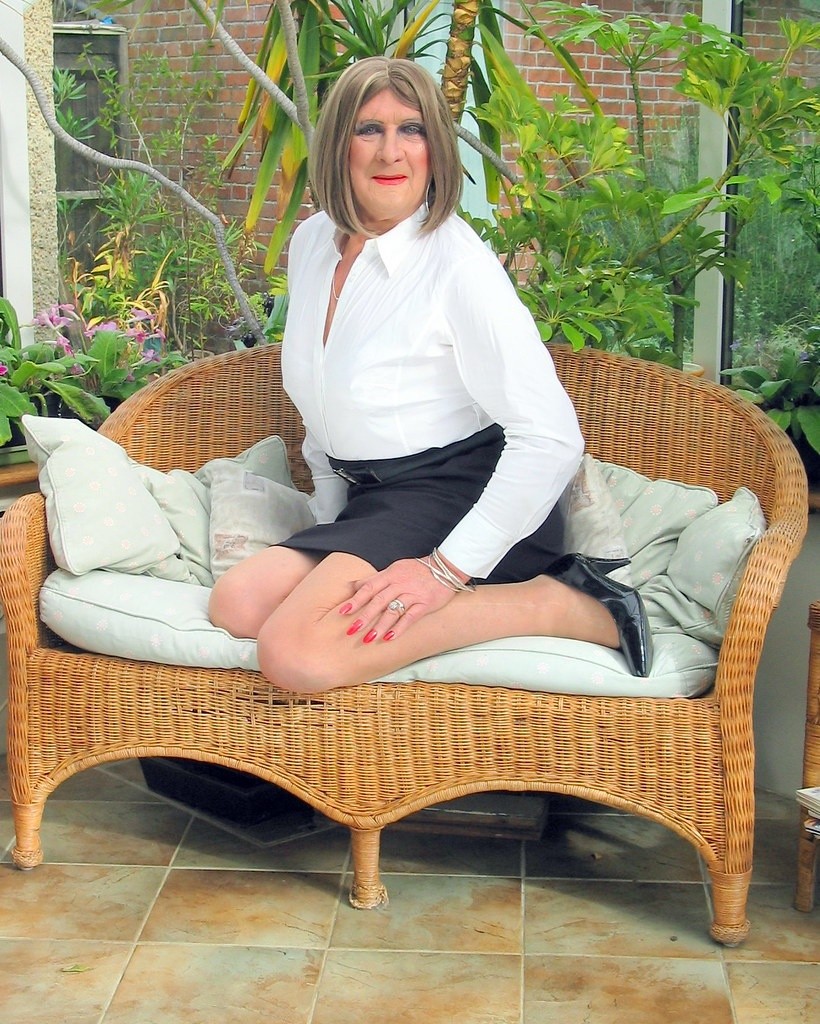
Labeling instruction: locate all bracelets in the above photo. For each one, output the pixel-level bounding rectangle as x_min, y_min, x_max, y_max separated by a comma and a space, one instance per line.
413, 545, 476, 595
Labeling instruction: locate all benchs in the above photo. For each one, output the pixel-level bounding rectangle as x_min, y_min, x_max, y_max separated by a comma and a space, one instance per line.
0, 339, 809, 947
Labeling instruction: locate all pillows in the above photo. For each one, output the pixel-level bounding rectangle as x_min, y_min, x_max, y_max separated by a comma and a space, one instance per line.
18, 413, 302, 590
203, 459, 320, 585
556, 450, 768, 652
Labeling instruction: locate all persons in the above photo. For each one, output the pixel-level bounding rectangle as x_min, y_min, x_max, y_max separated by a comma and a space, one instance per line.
204, 58, 650, 696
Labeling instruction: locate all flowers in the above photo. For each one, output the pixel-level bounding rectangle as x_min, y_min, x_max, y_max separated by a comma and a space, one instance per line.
25, 295, 179, 387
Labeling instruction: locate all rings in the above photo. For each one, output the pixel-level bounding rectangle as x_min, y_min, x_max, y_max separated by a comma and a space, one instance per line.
389, 599, 405, 616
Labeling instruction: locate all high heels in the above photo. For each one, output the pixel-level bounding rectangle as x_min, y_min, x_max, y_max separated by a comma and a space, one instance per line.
544, 553, 654, 677
